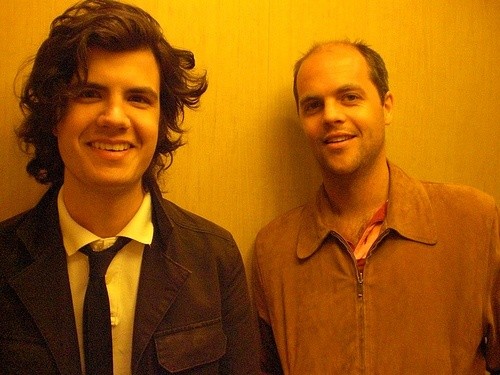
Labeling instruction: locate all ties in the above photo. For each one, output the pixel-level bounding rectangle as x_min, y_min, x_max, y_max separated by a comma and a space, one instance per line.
71, 237, 135, 375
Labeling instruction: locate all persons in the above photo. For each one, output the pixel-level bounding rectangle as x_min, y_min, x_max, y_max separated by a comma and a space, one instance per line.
0, 0, 266, 374
254, 41, 500, 374
